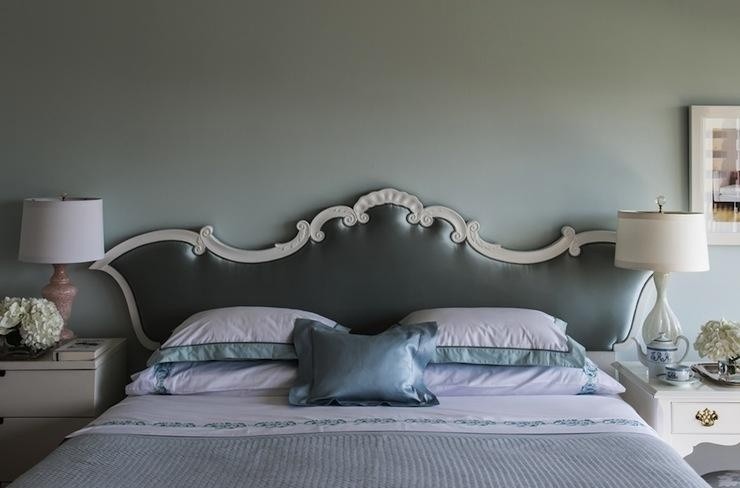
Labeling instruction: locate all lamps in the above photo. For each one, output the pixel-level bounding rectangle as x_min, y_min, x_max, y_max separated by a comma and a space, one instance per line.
616, 196, 711, 348
18, 192, 104, 342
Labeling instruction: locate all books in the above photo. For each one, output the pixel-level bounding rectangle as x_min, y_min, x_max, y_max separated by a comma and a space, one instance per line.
52, 337, 112, 360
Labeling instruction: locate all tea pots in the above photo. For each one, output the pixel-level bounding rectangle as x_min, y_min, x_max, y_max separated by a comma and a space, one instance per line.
630, 332, 690, 376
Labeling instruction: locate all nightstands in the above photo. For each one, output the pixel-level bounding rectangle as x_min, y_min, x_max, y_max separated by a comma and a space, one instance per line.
1, 335, 128, 486
609, 359, 740, 458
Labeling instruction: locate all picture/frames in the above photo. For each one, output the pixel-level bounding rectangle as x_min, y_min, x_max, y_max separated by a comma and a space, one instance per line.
690, 104, 740, 246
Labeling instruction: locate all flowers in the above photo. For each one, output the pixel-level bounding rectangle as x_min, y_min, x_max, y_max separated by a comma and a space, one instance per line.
0, 295, 66, 352
693, 319, 740, 367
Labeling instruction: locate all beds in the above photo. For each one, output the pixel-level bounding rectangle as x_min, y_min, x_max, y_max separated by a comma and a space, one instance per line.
7, 189, 710, 488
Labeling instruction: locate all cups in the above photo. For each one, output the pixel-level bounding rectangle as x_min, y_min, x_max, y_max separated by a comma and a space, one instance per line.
666, 365, 694, 381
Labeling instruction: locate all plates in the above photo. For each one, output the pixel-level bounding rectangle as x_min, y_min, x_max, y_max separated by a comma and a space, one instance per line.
655, 373, 702, 387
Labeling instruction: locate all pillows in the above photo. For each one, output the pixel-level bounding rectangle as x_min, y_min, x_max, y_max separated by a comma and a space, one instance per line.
145, 305, 352, 366
396, 306, 586, 369
288, 317, 439, 407
126, 355, 626, 397
